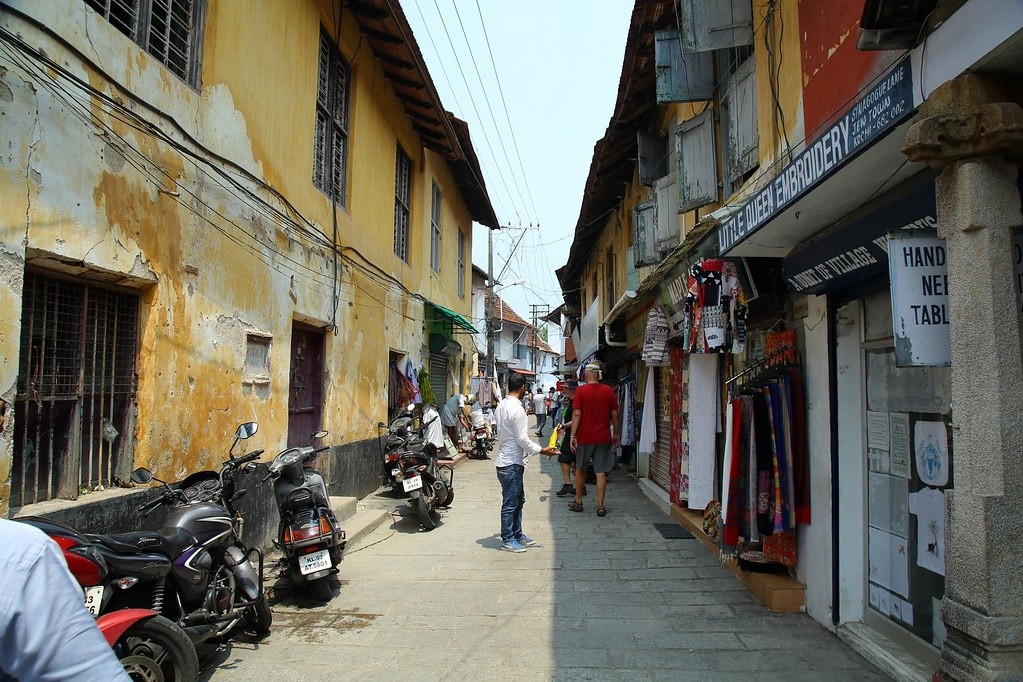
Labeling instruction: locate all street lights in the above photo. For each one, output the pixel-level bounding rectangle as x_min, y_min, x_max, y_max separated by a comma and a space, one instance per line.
488, 280, 525, 377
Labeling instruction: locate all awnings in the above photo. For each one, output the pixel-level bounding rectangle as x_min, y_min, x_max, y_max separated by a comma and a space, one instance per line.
427, 302, 481, 335
512, 369, 538, 375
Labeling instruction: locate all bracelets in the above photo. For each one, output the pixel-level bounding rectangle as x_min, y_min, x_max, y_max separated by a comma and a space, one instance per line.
570, 435, 575, 438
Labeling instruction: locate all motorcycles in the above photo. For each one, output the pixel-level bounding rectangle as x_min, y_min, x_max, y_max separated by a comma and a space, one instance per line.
466, 402, 496, 460
0, 422, 272, 682
399, 404, 454, 530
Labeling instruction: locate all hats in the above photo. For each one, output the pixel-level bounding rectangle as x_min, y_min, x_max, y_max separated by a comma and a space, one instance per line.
585, 364, 600, 373
561, 379, 578, 390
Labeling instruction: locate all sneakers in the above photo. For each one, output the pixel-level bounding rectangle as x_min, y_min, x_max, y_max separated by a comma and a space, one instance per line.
556, 483, 574, 495
500, 537, 527, 553
570, 486, 587, 496
516, 534, 536, 545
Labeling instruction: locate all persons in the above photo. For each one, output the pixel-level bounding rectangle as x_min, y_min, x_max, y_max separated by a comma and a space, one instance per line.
567, 364, 621, 518
548, 386, 558, 429
532, 388, 552, 438
442, 394, 476, 453
554, 378, 588, 496
0, 518, 132, 682
492, 373, 560, 552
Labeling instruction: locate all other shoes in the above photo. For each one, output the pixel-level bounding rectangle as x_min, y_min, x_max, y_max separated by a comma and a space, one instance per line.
535, 432, 545, 437
549, 426, 554, 429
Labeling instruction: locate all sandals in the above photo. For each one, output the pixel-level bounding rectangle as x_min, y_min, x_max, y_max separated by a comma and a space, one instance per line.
597, 505, 606, 517
458, 448, 468, 453
568, 499, 584, 513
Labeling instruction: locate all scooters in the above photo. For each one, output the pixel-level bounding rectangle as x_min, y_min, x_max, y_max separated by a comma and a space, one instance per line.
242, 430, 347, 601
378, 404, 416, 498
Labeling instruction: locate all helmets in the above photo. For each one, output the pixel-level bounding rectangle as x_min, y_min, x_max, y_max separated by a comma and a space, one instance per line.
466, 394, 476, 405
